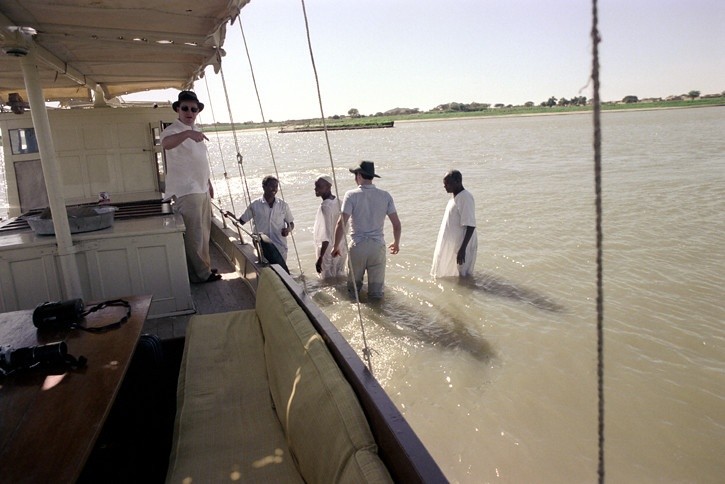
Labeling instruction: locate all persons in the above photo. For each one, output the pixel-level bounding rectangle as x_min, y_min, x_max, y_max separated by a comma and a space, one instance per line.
160, 91, 222, 284
331, 159, 401, 297
224, 175, 294, 265
430, 169, 478, 278
314, 174, 350, 278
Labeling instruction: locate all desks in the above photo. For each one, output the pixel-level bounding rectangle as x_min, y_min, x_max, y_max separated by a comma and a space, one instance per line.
0, 294, 155, 484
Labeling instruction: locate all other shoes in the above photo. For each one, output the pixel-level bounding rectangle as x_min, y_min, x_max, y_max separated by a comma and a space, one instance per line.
211, 268, 218, 274
201, 273, 222, 282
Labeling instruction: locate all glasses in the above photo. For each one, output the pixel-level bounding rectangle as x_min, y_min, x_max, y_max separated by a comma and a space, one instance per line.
178, 105, 199, 113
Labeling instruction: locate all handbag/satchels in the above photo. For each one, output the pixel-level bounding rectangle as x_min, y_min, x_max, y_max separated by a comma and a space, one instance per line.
32, 297, 84, 328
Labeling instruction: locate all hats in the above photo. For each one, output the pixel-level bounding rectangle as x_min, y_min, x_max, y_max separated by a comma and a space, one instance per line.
172, 90, 205, 113
348, 160, 381, 178
320, 174, 333, 186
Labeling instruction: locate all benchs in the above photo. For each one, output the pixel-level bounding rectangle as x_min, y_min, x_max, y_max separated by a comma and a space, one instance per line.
162, 266, 396, 484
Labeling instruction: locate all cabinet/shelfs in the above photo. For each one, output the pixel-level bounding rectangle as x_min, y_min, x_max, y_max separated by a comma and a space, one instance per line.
0, 211, 197, 322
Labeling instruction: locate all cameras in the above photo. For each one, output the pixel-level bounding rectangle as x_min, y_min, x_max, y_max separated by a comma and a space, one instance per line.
0, 339, 67, 385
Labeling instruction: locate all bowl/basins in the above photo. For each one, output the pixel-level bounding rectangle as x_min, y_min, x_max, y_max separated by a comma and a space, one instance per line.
23, 206, 119, 235
